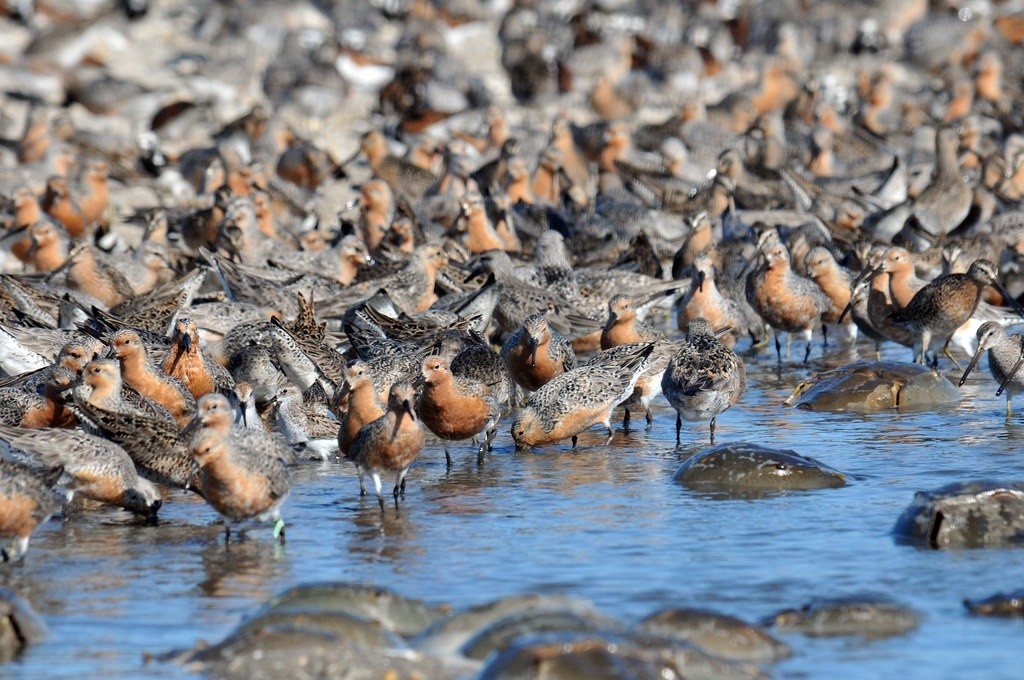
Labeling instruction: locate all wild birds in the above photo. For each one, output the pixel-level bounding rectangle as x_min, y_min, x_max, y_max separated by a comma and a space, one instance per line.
0, 0, 1024, 566
